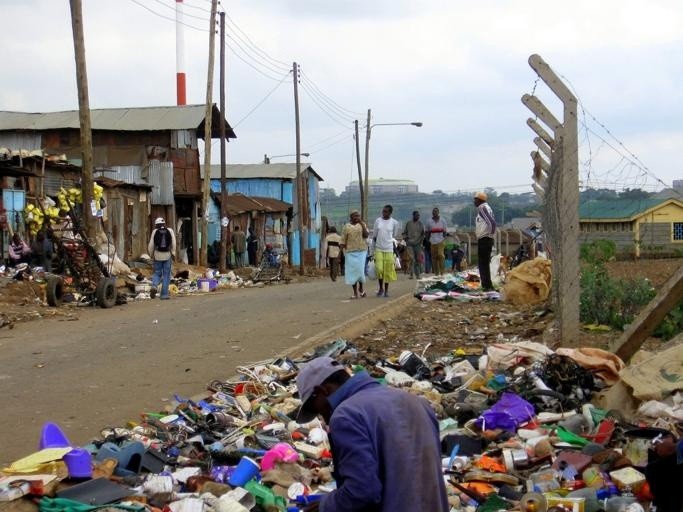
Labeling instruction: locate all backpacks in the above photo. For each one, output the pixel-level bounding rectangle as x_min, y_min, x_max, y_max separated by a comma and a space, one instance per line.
154, 227, 173, 251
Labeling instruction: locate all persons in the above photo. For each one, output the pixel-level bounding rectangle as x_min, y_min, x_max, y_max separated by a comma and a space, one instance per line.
324, 204, 465, 299
293, 355, 450, 511
8, 222, 52, 269
472, 191, 496, 292
147, 217, 176, 300
208, 224, 258, 269
261, 244, 274, 260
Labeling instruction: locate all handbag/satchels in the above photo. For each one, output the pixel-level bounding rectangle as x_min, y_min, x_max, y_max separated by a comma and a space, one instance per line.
395, 257, 401, 270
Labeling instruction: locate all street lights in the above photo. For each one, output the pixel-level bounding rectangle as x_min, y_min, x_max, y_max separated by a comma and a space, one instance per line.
362, 109, 425, 220
263, 152, 310, 162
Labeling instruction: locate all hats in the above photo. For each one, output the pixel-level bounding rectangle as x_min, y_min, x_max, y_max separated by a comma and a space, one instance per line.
295, 357, 346, 423
475, 192, 488, 202
155, 218, 165, 224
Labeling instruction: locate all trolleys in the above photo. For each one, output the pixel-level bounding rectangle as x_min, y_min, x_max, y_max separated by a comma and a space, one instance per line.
510, 228, 545, 269
33, 193, 128, 307
253, 249, 285, 282
